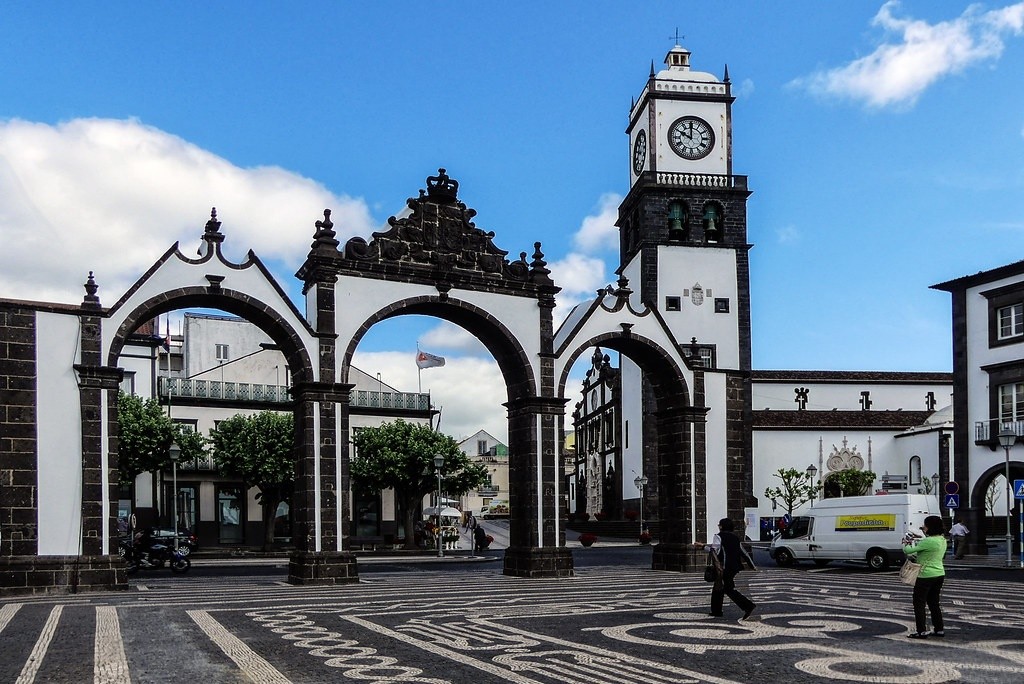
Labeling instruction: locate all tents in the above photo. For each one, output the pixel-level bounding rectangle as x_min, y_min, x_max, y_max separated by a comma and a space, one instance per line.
422, 505, 462, 527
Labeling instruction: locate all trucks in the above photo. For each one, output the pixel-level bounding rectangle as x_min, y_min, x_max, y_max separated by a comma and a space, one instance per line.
481, 499, 510, 520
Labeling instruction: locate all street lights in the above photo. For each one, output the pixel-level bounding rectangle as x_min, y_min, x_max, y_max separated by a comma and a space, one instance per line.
996, 426, 1018, 566
634, 474, 648, 535
434, 453, 445, 557
169, 442, 182, 550
806, 464, 818, 508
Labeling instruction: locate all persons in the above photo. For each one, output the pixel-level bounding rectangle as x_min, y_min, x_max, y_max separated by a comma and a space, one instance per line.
463, 513, 474, 534
474, 523, 486, 552
695, 518, 757, 620
952, 518, 969, 560
116, 516, 128, 533
901, 515, 948, 638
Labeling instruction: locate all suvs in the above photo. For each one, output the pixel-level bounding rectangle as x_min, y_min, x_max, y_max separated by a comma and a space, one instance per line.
118, 526, 200, 558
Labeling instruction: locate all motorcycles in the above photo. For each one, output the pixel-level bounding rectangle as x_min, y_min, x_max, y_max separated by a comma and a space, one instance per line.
122, 540, 189, 574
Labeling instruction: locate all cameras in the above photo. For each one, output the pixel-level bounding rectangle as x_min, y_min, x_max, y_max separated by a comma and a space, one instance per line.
906, 533, 913, 540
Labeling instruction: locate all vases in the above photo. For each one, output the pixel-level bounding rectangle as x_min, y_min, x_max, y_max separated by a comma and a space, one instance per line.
641, 538, 651, 545
581, 541, 593, 546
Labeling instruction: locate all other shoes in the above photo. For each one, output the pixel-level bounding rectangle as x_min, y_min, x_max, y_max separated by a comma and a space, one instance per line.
709, 611, 724, 618
908, 631, 928, 638
743, 602, 756, 620
929, 629, 946, 637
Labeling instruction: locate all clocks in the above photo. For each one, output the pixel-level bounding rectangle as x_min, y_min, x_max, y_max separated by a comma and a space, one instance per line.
667, 115, 715, 160
633, 128, 646, 177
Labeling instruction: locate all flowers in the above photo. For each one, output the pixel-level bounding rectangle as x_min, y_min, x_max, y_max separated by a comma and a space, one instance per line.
639, 530, 656, 539
624, 509, 640, 518
581, 512, 590, 519
486, 535, 494, 542
594, 512, 608, 520
578, 533, 599, 543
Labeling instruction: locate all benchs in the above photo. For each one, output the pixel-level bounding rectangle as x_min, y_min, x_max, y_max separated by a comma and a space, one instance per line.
351, 536, 385, 552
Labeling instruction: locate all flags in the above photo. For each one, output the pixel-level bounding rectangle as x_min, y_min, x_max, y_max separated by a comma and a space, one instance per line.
415, 350, 445, 368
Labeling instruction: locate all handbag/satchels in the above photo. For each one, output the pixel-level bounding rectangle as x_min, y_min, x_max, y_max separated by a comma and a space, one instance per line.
704, 547, 718, 582
898, 557, 922, 586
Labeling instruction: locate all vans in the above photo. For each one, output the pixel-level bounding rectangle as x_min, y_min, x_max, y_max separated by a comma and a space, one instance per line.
272, 521, 291, 550
769, 495, 942, 571
